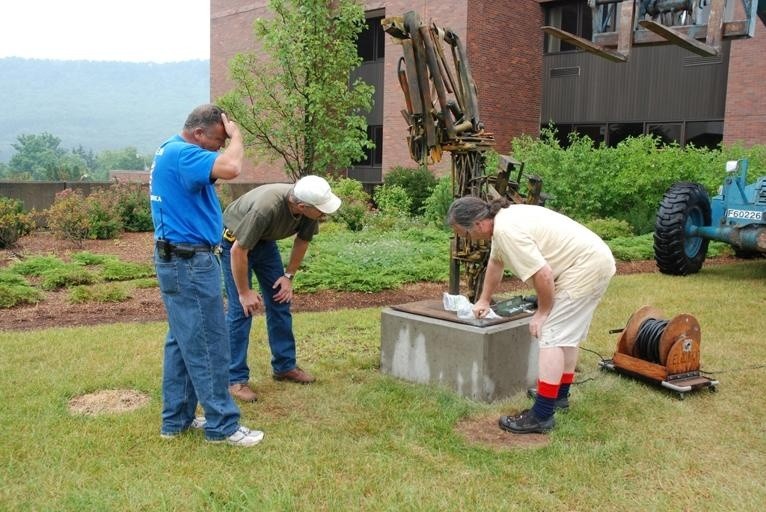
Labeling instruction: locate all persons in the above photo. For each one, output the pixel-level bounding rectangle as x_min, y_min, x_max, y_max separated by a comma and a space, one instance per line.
149, 105, 264, 446
447, 195, 617, 434
219, 175, 342, 403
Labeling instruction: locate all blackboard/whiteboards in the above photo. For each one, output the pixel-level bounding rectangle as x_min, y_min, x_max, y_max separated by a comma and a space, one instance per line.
538, 1, 766, 276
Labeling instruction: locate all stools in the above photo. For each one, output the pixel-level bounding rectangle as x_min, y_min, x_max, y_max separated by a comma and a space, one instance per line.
170, 244, 222, 256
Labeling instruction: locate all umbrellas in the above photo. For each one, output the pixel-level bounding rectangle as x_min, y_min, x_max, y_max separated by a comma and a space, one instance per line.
272, 367, 316, 385
227, 383, 258, 403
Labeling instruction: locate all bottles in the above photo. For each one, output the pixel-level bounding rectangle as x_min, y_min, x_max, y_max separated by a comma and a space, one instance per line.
294, 174, 341, 214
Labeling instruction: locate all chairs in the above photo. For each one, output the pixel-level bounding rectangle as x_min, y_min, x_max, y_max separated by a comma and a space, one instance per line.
205, 425, 265, 448
526, 388, 571, 409
498, 409, 556, 434
160, 416, 207, 439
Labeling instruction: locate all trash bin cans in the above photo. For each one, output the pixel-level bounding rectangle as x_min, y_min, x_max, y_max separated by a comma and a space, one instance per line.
284, 272, 294, 280
